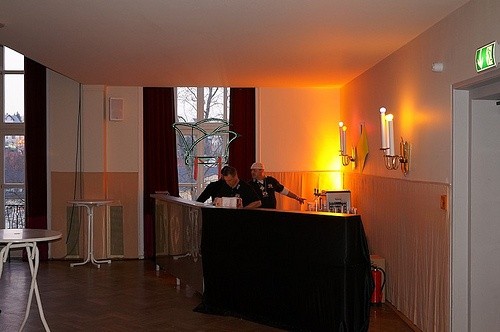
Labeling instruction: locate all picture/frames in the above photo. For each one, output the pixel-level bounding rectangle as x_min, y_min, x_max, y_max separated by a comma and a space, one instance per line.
110, 97, 124, 120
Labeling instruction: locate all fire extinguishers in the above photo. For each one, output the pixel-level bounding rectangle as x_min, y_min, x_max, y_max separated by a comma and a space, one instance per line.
371, 264, 385, 306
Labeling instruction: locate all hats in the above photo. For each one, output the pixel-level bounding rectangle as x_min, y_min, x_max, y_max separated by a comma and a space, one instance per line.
250, 163, 265, 169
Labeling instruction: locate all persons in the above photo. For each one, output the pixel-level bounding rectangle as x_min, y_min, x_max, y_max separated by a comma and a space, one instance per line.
247, 163, 306, 209
196, 166, 261, 209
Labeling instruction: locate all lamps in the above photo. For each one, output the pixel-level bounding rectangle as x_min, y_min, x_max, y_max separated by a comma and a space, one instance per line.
338, 123, 357, 166
380, 106, 410, 175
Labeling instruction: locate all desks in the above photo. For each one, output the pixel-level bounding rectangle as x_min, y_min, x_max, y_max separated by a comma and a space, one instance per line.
0, 228, 62, 332
68, 200, 113, 268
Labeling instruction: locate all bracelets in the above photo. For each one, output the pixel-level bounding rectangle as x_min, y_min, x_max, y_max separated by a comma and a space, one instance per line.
296, 195, 300, 200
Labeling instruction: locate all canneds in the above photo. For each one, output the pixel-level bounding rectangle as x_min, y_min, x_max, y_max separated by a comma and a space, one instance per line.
236, 198, 243, 209
